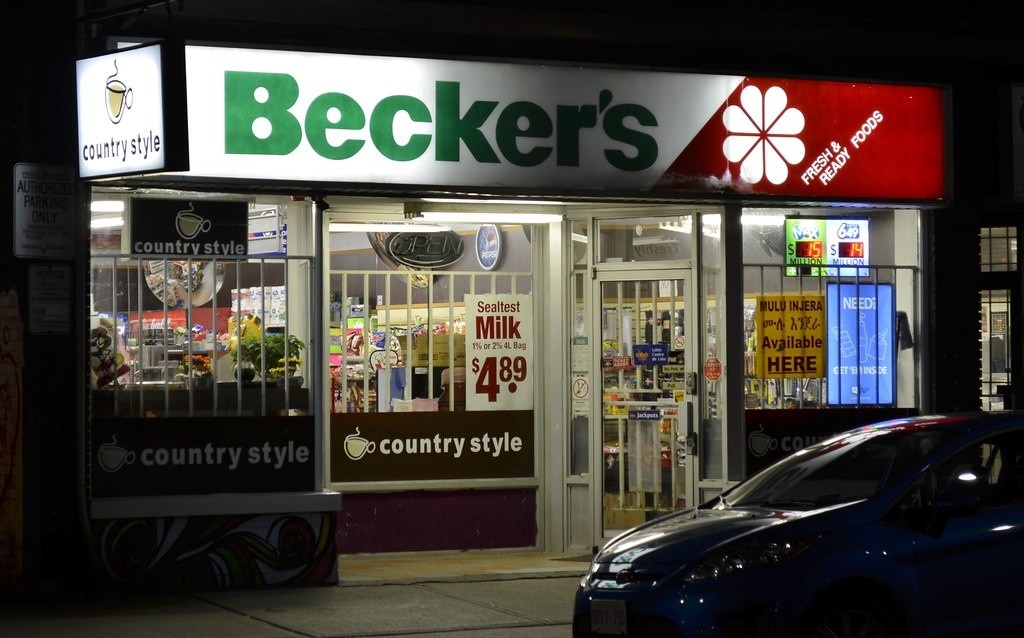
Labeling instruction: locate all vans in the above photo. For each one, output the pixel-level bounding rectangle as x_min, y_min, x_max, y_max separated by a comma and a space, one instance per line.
571, 410, 1024, 638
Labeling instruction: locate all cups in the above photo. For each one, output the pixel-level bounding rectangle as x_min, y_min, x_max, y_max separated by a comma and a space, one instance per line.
176, 213, 211, 240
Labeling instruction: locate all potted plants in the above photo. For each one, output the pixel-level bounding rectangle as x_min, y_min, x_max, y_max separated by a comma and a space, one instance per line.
231, 333, 260, 382
242, 334, 305, 382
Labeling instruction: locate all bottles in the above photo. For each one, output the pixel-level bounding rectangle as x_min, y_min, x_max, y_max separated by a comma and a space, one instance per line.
437, 368, 466, 412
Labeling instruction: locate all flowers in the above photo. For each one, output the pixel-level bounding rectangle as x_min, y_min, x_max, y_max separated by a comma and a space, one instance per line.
174, 355, 213, 379
271, 356, 303, 379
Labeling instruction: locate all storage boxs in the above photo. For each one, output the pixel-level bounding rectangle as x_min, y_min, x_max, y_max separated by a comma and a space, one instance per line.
603, 492, 645, 529
145, 329, 174, 345
396, 333, 466, 367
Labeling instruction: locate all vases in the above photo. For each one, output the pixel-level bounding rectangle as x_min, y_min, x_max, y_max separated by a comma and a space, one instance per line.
277, 376, 305, 388
185, 377, 212, 390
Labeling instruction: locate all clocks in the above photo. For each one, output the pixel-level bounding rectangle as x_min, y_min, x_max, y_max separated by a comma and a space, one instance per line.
475, 224, 502, 270
